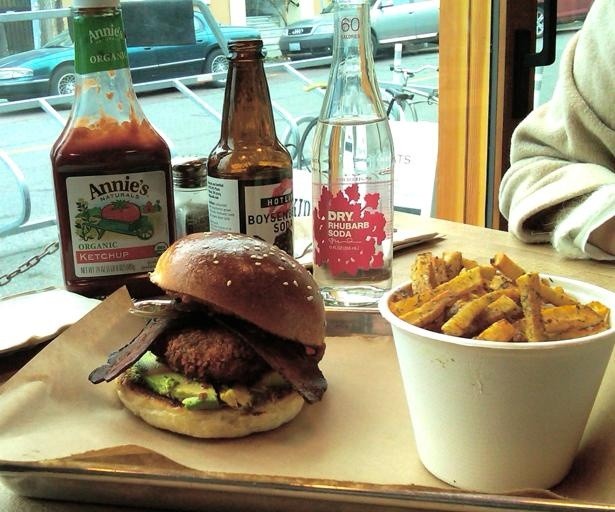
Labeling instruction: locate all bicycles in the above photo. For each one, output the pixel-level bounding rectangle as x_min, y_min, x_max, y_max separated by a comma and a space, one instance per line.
286, 64, 441, 171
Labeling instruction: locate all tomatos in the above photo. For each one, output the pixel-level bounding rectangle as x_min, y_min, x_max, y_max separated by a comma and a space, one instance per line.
102, 202, 141, 222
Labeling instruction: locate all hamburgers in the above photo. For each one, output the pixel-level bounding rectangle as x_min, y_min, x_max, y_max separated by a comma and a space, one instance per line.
87, 230, 325, 438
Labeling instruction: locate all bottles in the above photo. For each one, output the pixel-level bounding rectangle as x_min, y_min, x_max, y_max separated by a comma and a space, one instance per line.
204, 37, 294, 259
47, 0, 177, 301
170, 155, 209, 240
308, 0, 395, 310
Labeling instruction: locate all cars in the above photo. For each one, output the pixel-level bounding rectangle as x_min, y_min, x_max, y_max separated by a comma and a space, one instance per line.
2, 10, 268, 111
278, 1, 439, 62
533, 1, 593, 38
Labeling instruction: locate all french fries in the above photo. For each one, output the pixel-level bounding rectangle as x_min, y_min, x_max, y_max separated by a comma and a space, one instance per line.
390, 250, 611, 342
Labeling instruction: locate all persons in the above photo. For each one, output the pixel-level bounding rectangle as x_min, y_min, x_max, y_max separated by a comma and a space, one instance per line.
497, 1, 615, 265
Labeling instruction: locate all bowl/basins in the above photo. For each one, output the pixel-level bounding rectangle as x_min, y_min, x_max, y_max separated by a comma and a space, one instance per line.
378, 272, 615, 496
